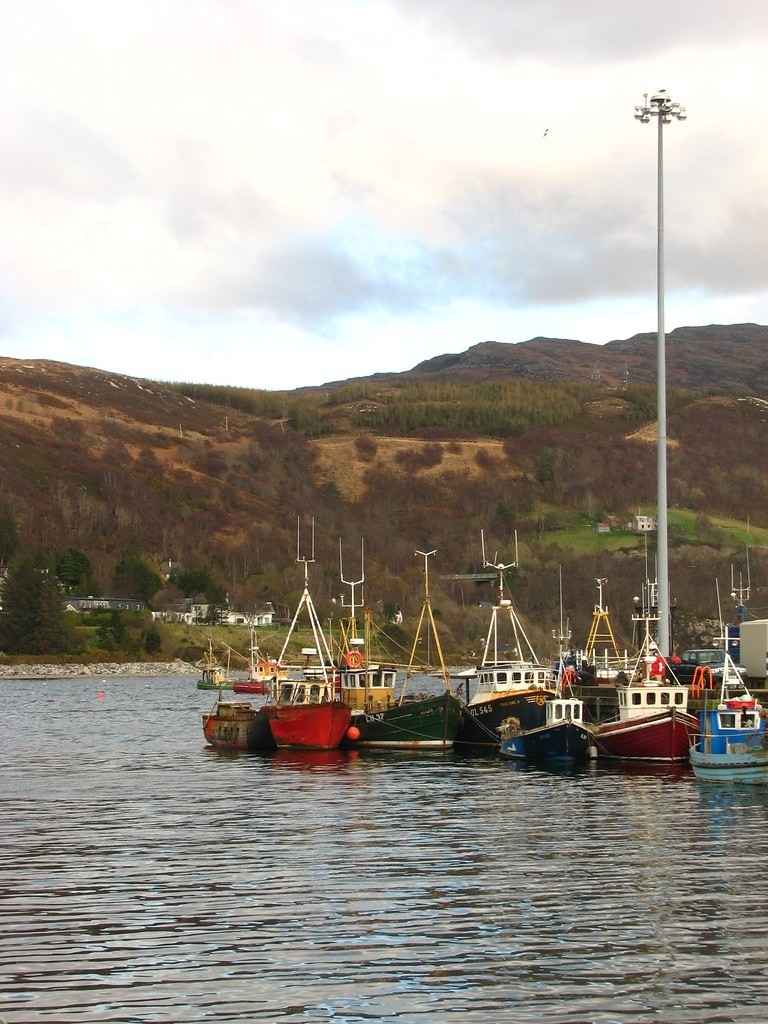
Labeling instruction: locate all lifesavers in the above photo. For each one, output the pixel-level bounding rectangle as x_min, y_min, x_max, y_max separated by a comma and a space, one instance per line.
633, 680, 666, 687
345, 650, 362, 669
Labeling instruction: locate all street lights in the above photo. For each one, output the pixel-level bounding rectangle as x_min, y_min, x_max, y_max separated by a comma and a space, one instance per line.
635, 86, 690, 659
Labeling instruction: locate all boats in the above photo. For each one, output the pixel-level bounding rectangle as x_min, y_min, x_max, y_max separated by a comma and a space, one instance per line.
192, 515, 768, 785
